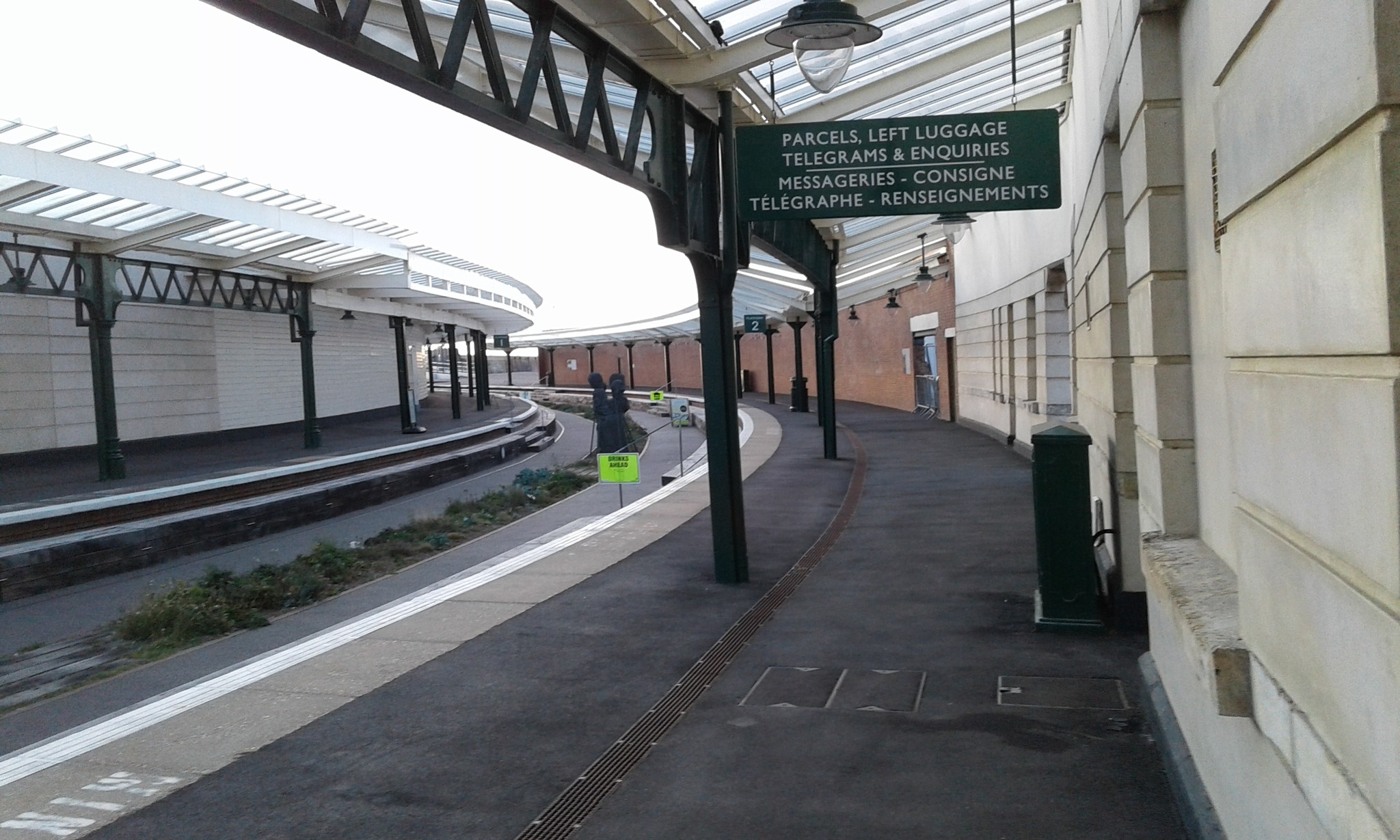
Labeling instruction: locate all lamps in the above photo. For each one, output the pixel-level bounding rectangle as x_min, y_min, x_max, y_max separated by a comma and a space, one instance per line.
912, 232, 935, 294
931, 212, 977, 243
847, 304, 861, 327
5, 233, 37, 300
405, 318, 446, 348
765, 0, 882, 94
883, 288, 901, 316
340, 310, 358, 326
